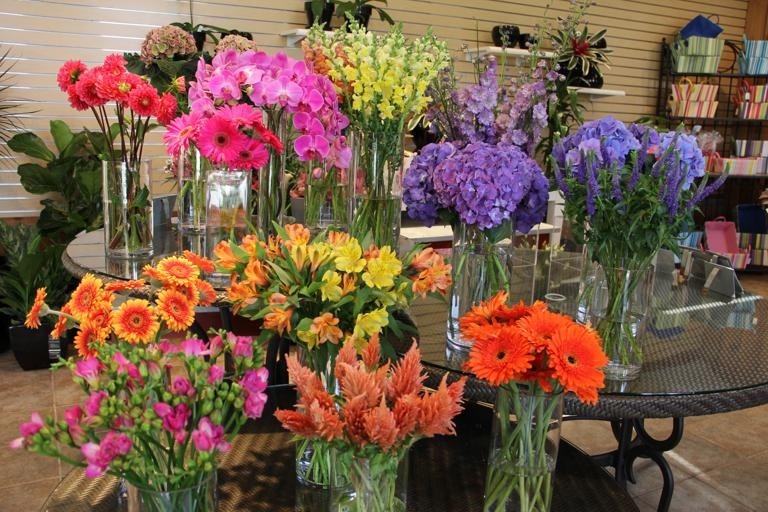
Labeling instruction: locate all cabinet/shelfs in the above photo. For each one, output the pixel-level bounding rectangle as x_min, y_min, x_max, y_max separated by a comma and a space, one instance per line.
653, 34, 768, 276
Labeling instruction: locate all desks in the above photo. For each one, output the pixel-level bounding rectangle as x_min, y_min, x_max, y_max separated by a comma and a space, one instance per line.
60, 222, 768, 512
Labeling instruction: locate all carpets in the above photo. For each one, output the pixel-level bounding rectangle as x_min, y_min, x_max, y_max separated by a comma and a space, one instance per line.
634, 275, 768, 512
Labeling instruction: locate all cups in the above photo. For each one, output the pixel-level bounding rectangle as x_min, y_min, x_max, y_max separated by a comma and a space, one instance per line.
519, 32, 538, 50
492, 24, 520, 48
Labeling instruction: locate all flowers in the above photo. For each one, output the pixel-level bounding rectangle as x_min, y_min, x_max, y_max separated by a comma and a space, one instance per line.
1, 1, 734, 512
